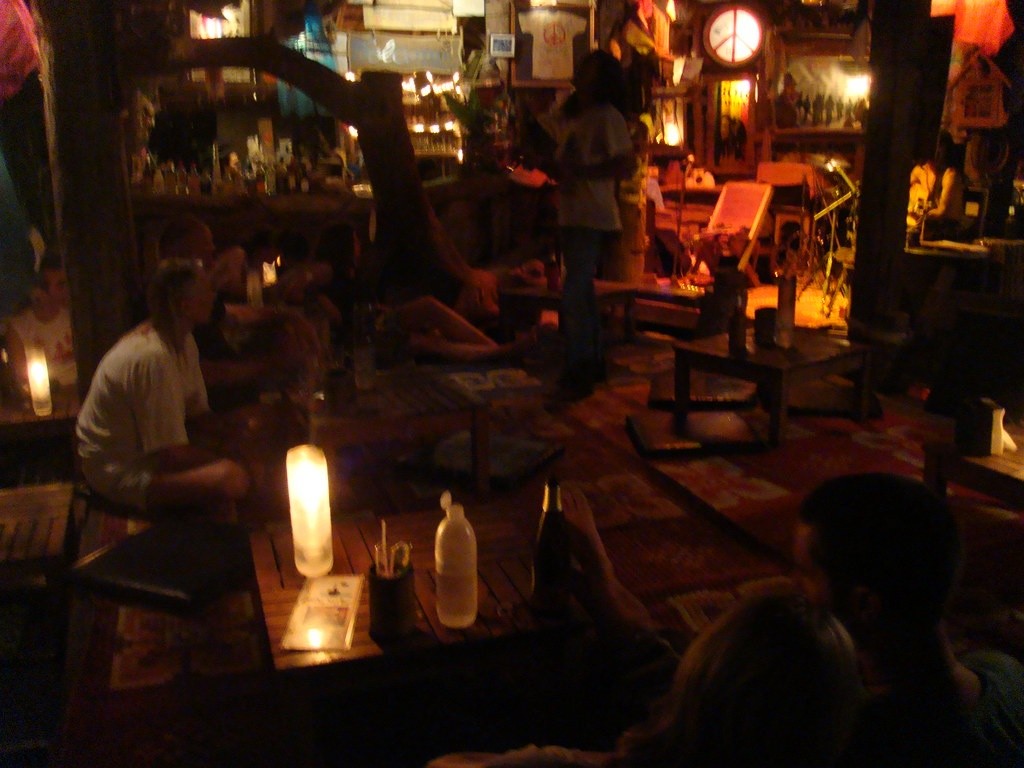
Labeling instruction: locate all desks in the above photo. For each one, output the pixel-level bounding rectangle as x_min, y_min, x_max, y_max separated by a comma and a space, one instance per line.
0, 382, 78, 487
0, 481, 77, 603
921, 421, 1024, 514
498, 275, 635, 371
671, 322, 874, 449
249, 503, 596, 768
270, 363, 491, 516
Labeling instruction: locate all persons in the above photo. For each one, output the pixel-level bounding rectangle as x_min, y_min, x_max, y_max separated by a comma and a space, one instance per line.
925, 131, 965, 220
424, 470, 1024, 768
524, 49, 637, 400
76, 215, 535, 523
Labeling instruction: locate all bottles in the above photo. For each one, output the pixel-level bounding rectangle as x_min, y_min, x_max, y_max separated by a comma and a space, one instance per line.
154, 168, 164, 195
531, 477, 565, 618
165, 158, 288, 197
352, 300, 376, 388
435, 492, 477, 628
728, 306, 746, 352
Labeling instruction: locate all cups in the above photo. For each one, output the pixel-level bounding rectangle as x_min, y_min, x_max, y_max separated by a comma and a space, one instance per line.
368, 560, 414, 642
755, 307, 775, 347
